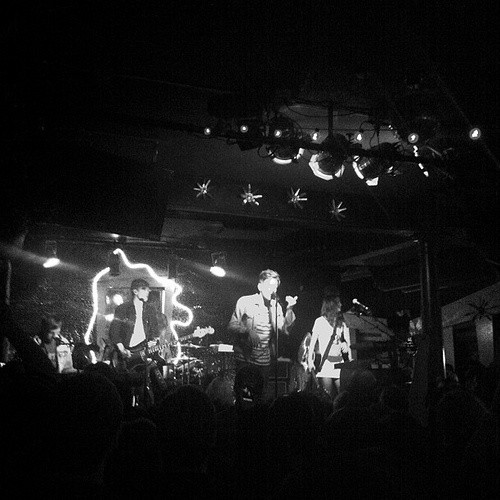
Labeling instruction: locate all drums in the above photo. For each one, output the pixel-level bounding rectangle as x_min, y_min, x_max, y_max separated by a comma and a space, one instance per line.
177, 361, 196, 376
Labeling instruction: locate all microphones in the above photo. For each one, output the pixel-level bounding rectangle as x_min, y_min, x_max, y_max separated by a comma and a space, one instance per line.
271, 292, 278, 299
352, 298, 369, 310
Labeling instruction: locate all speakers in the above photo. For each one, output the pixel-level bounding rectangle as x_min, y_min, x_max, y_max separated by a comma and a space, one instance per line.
34, 144, 169, 242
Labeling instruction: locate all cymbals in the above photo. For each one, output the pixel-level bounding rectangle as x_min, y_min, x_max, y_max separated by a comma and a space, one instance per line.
172, 342, 205, 349
170, 355, 197, 360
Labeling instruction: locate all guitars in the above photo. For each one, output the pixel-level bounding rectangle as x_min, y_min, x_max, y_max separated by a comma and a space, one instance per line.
111, 325, 215, 376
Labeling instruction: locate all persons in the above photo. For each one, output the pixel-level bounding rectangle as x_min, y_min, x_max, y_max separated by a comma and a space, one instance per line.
0, 313, 500, 500
110, 279, 168, 405
309, 292, 351, 398
228, 270, 298, 406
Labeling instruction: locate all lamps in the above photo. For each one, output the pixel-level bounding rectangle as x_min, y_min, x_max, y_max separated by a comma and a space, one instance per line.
209, 250, 229, 278
108, 247, 121, 276
41, 240, 61, 268
201, 101, 483, 186
